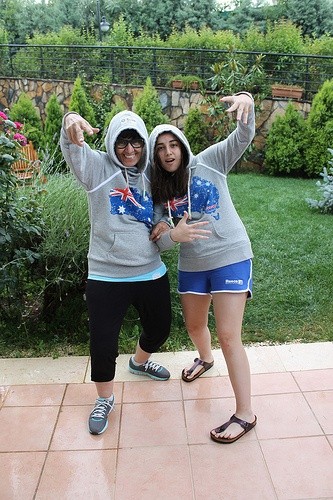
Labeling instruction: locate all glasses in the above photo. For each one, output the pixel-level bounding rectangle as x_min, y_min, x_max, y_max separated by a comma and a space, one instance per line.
114, 137, 144, 150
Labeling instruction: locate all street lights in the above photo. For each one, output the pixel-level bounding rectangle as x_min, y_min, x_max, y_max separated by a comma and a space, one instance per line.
96, 0, 111, 83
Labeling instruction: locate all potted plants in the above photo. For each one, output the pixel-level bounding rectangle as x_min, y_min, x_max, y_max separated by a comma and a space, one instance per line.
272, 81, 305, 101
165, 75, 203, 89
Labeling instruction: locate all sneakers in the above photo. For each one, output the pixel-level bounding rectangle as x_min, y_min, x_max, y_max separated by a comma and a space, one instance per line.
88, 393, 114, 435
127, 356, 170, 381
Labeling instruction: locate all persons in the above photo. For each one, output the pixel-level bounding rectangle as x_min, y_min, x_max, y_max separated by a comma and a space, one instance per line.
60, 110, 171, 435
149, 92, 257, 444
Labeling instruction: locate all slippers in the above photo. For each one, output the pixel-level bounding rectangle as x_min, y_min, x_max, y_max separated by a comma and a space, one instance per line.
180, 358, 215, 382
210, 413, 257, 444
